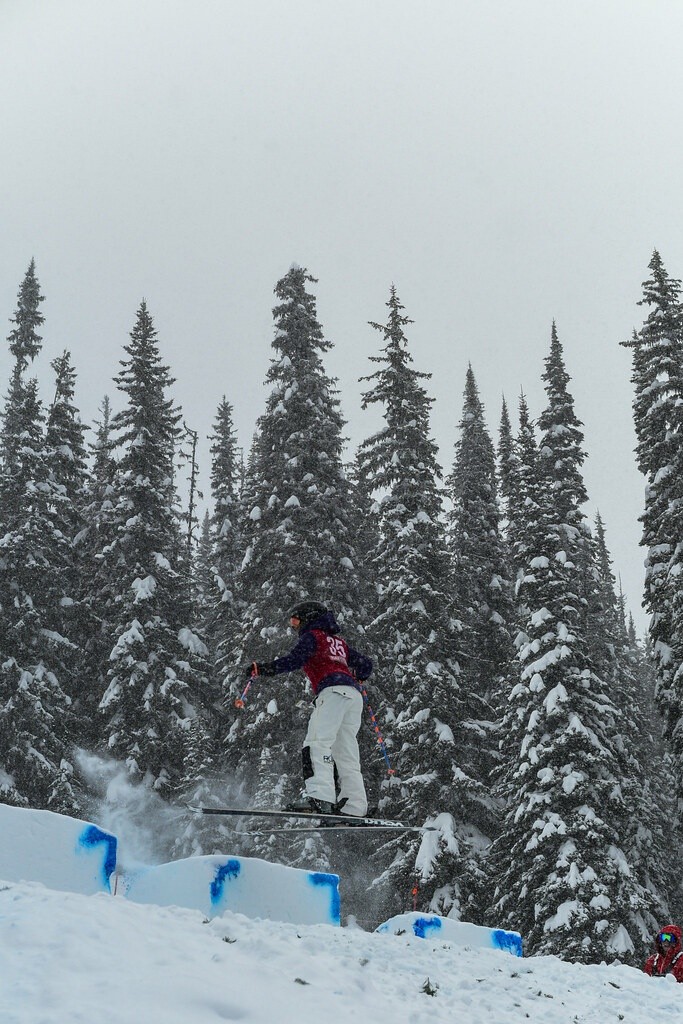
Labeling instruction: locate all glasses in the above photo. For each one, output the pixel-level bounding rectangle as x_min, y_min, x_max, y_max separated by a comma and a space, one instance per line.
659, 933, 676, 942
289, 616, 300, 626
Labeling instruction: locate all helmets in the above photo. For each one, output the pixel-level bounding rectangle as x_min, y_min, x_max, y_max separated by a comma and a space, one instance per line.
291, 601, 327, 622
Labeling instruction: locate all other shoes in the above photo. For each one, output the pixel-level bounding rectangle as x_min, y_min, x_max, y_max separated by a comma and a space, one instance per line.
293, 797, 333, 814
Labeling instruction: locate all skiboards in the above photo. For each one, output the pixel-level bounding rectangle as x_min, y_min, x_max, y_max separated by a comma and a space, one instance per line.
183, 800, 442, 838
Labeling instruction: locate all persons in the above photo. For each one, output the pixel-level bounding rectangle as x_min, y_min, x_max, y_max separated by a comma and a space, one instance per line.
643, 924, 683, 983
246, 601, 373, 817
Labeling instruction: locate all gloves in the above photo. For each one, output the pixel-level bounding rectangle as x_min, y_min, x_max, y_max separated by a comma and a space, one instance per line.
351, 668, 370, 681
246, 663, 270, 677
